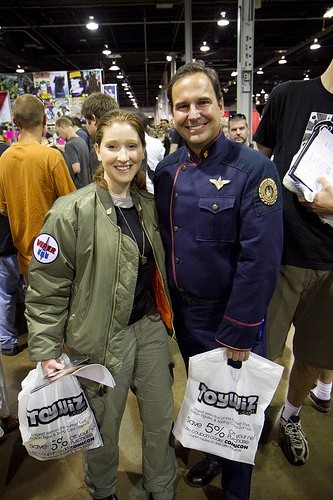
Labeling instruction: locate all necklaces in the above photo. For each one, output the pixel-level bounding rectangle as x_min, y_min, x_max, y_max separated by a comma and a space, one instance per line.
109, 191, 148, 266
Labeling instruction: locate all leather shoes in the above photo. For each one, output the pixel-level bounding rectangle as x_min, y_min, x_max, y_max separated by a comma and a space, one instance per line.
185, 457, 222, 488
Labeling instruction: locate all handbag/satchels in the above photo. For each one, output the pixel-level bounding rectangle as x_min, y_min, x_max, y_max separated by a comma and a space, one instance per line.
172, 347, 284, 467
18, 355, 103, 460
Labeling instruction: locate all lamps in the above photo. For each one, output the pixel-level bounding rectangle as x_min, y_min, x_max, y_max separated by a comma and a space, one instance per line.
200, 41, 210, 51
231, 68, 237, 77
85, 10, 98, 30
252, 88, 269, 105
108, 60, 120, 70
310, 38, 321, 50
15, 64, 25, 73
116, 72, 124, 79
122, 81, 139, 109
166, 53, 173, 62
303, 72, 310, 80
278, 55, 287, 64
102, 39, 111, 55
217, 11, 230, 26
256, 67, 264, 75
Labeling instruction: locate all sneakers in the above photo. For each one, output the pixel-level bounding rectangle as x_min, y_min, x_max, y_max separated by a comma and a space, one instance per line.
307, 384, 332, 413
277, 407, 310, 466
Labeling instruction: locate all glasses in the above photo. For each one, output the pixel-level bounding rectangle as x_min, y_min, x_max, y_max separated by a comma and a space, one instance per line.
228, 113, 248, 127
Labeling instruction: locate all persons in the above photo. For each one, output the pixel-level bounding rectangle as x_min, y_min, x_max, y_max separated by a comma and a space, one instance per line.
0, 59, 333, 500
151, 59, 282, 500
24, 110, 178, 500
32, 81, 52, 98
